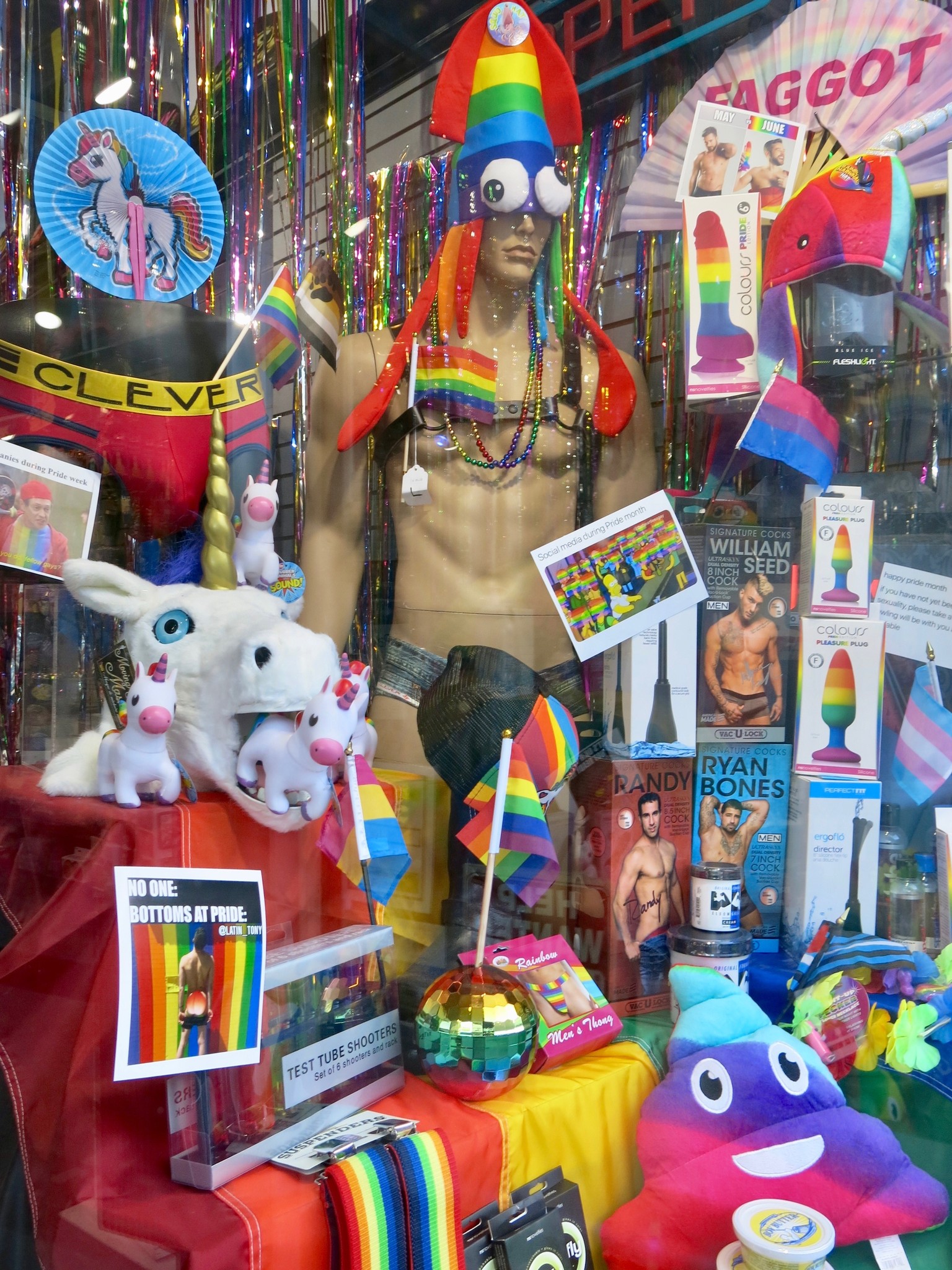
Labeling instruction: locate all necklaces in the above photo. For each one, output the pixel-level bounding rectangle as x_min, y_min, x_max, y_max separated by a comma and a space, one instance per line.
427, 290, 542, 470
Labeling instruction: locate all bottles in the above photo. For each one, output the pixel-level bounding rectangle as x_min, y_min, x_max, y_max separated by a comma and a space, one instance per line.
874, 802, 939, 951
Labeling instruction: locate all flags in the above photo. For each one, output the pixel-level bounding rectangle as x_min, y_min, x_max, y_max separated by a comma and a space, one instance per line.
734, 374, 840, 497
790, 920, 917, 992
456, 692, 579, 909
316, 754, 412, 906
892, 660, 952, 806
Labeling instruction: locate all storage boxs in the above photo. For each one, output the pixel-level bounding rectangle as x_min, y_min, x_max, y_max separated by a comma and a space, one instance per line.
794, 480, 882, 619
458, 1164, 595, 1270
795, 265, 893, 392
791, 617, 885, 776
162, 922, 401, 1194
694, 741, 795, 954
682, 190, 758, 416
567, 756, 694, 1017
456, 934, 623, 1074
682, 521, 795, 742
786, 769, 883, 946
597, 607, 698, 758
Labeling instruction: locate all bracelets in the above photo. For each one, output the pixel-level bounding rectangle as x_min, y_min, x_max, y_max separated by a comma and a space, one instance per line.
775, 694, 782, 699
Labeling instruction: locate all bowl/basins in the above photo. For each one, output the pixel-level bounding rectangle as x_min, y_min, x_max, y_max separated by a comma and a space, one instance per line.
717, 1198, 837, 1270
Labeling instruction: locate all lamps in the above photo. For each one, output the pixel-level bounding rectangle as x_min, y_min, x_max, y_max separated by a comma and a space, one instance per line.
90, 57, 132, 106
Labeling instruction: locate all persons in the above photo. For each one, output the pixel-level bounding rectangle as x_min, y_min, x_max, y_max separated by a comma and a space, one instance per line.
302, 0, 654, 974
704, 574, 783, 724
733, 138, 789, 213
698, 796, 769, 933
0, 472, 68, 578
175, 928, 215, 1059
613, 792, 685, 997
689, 127, 737, 197
518, 961, 594, 1027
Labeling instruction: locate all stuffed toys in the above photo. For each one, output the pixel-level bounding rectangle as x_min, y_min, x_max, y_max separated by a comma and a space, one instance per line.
600, 965, 950, 1270
37, 409, 343, 832
